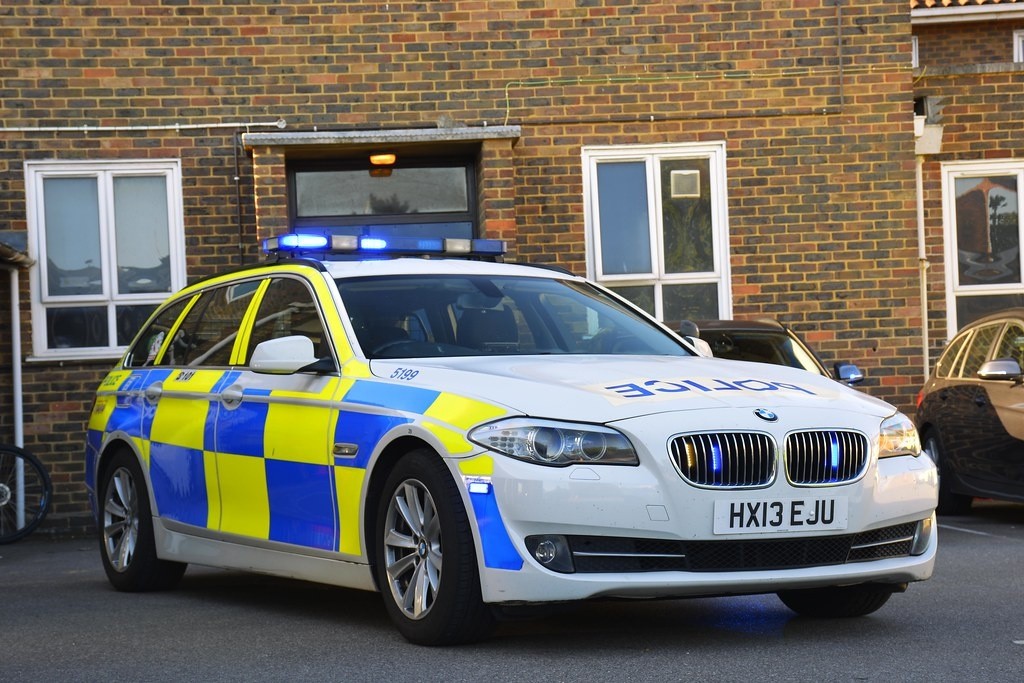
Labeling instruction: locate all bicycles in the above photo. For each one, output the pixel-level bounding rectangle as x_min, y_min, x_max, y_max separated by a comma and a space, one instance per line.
0, 443, 52, 545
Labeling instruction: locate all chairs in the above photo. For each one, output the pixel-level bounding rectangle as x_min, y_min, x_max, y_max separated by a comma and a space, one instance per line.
317, 289, 409, 361
460, 302, 521, 351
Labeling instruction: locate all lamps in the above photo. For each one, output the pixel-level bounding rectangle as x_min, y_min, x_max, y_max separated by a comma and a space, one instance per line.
368, 169, 391, 177
369, 148, 397, 164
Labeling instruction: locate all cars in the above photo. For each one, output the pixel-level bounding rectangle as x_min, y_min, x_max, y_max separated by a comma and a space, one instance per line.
914, 307, 1024, 513
591, 317, 871, 392
85, 231, 940, 649
